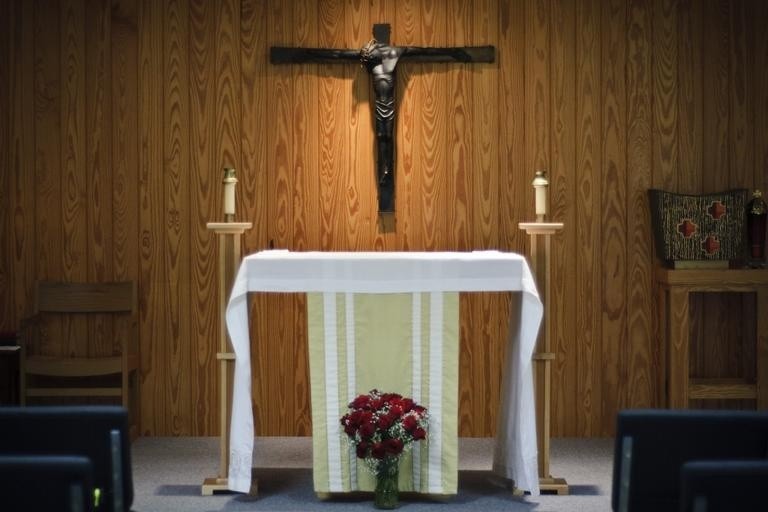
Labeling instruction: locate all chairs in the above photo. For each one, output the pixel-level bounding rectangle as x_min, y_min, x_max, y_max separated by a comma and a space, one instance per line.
18, 274, 145, 436
0, 400, 136, 511
611, 410, 768, 512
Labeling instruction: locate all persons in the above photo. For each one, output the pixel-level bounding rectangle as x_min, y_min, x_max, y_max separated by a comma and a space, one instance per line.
297, 39, 471, 187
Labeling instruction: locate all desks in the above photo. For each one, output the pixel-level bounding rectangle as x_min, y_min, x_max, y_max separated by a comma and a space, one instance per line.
650, 264, 768, 414
222, 248, 533, 497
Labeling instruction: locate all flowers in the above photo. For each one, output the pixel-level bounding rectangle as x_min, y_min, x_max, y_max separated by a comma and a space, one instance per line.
339, 386, 429, 477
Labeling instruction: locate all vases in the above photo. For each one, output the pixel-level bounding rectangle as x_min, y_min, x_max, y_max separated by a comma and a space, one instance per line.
370, 448, 399, 508
